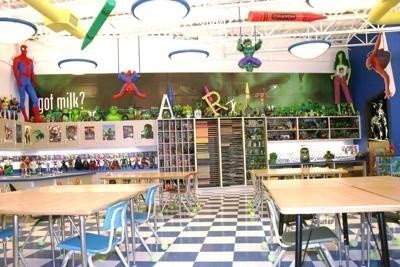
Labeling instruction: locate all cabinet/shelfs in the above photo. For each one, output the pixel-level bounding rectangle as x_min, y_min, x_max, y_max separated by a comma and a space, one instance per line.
157, 117, 198, 191
266, 115, 362, 140
241, 117, 267, 186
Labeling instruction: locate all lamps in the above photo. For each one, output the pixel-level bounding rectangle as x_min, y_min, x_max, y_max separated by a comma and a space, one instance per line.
287, 38, 332, 59
0, 17, 37, 45
58, 58, 99, 75
131, 0, 191, 24
167, 48, 209, 63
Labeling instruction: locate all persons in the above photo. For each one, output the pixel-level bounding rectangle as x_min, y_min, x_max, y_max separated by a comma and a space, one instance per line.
323, 151, 335, 162
0, 152, 157, 178
365, 32, 391, 101
299, 148, 310, 162
236, 38, 262, 72
330, 51, 357, 116
13, 45, 48, 123
4, 124, 154, 143
113, 69, 147, 101
0, 96, 193, 121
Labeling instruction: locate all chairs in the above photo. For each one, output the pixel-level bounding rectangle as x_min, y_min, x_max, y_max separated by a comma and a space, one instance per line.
0, 168, 202, 267
249, 166, 400, 267
